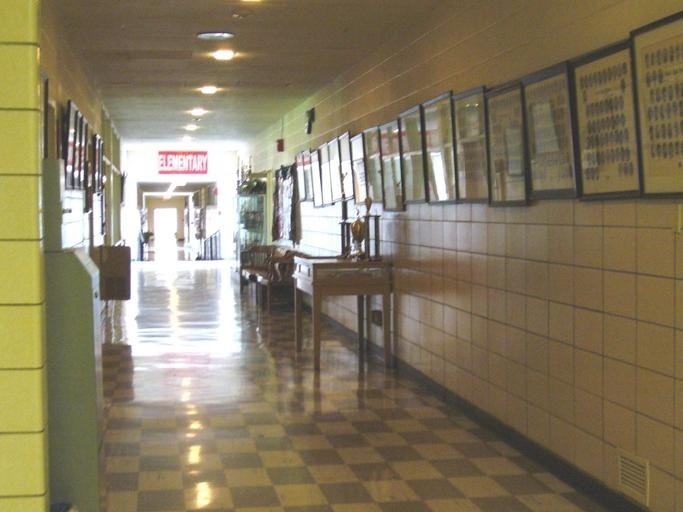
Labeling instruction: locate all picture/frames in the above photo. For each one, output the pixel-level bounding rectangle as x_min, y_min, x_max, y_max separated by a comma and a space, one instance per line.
451, 85, 489, 205
397, 105, 427, 207
484, 77, 531, 208
289, 118, 406, 212
570, 39, 641, 202
420, 90, 458, 206
62, 94, 105, 192
519, 61, 580, 202
632, 11, 683, 200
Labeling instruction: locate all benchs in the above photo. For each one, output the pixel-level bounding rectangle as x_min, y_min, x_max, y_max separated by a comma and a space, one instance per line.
237, 244, 305, 320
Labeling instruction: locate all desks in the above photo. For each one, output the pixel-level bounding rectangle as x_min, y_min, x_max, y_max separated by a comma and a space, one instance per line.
287, 250, 400, 374
